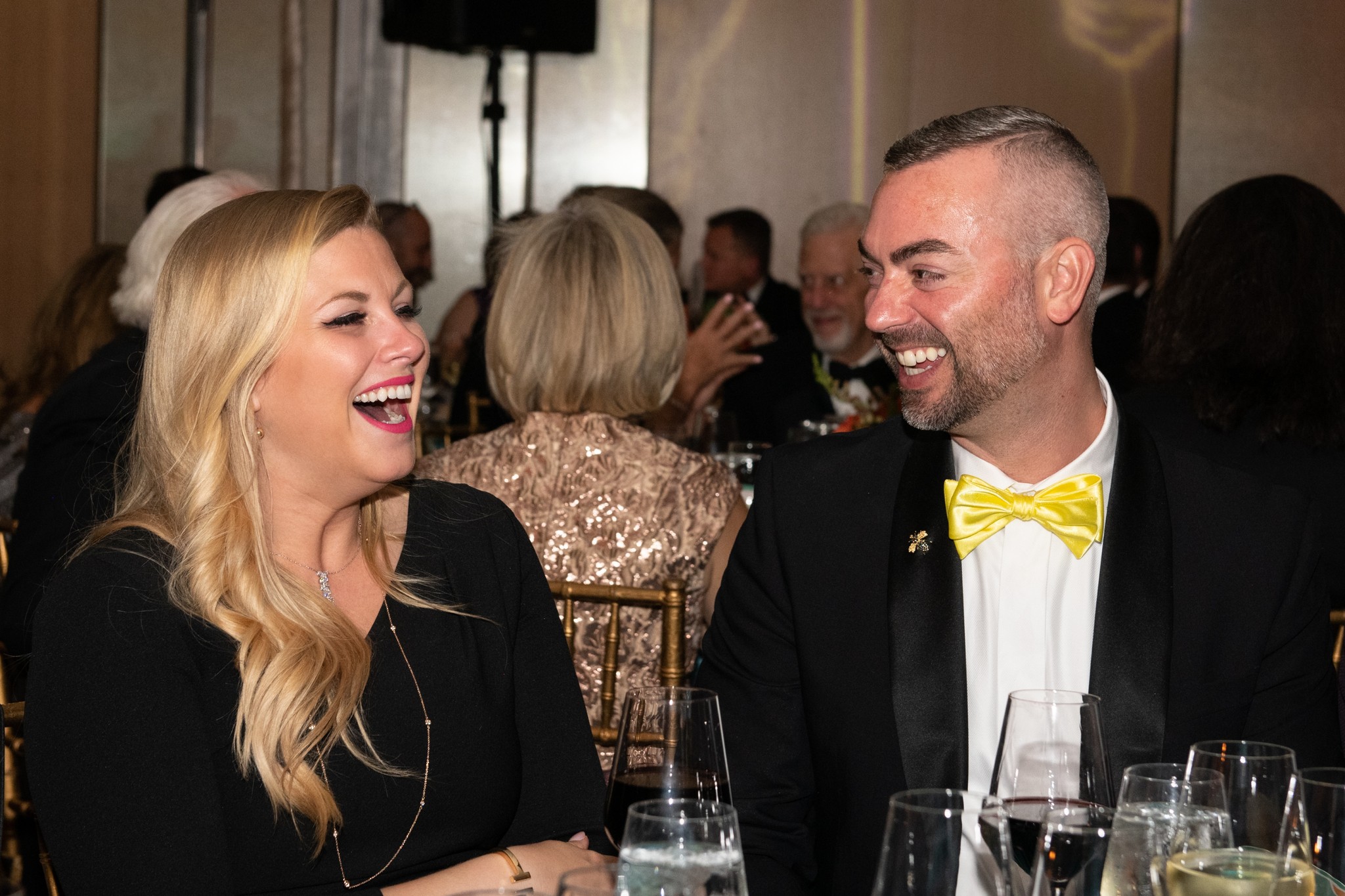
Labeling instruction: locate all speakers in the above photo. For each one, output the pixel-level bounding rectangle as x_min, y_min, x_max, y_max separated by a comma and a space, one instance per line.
377, 1, 597, 58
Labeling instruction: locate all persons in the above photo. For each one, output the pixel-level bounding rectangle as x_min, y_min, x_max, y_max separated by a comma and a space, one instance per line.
714, 201, 894, 449
2, 163, 1171, 679
1124, 172, 1344, 518
408, 196, 750, 794
23, 182, 614, 896
686, 104, 1345, 896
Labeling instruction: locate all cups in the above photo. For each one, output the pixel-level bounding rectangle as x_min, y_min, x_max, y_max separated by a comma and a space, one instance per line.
601, 680, 1345, 896
715, 443, 770, 507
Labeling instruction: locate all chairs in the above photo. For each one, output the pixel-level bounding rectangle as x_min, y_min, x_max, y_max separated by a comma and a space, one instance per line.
546, 574, 708, 779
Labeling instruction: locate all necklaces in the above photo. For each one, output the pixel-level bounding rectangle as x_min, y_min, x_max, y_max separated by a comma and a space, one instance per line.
269, 508, 432, 890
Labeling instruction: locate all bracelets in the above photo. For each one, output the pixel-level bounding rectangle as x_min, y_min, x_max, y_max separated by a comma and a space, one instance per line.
496, 847, 534, 896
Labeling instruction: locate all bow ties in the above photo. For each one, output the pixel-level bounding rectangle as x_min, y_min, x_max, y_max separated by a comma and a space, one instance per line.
943, 473, 1107, 560
829, 356, 898, 402
